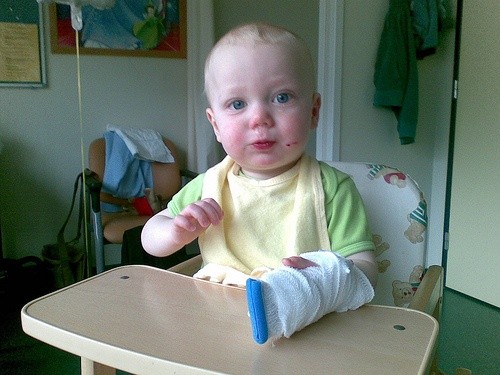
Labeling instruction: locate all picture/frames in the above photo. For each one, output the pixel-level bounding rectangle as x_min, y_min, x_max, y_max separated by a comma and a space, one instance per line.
46, 0, 188, 58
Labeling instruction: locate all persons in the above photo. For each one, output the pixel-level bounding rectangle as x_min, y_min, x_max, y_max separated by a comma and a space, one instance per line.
141, 22, 379, 344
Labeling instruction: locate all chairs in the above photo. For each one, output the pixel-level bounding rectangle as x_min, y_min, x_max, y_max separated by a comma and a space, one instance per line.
21, 161, 443, 375
84, 137, 198, 275
